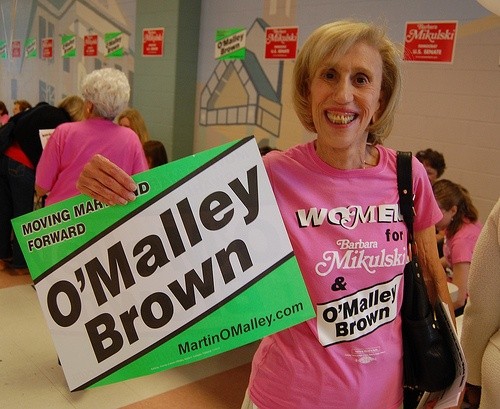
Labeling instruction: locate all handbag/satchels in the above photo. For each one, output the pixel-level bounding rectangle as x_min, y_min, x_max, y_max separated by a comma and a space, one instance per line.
397, 149, 457, 392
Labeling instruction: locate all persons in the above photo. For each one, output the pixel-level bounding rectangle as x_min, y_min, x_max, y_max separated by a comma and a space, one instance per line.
0, 69, 167, 366
414, 147, 500, 409
75, 21, 465, 409
